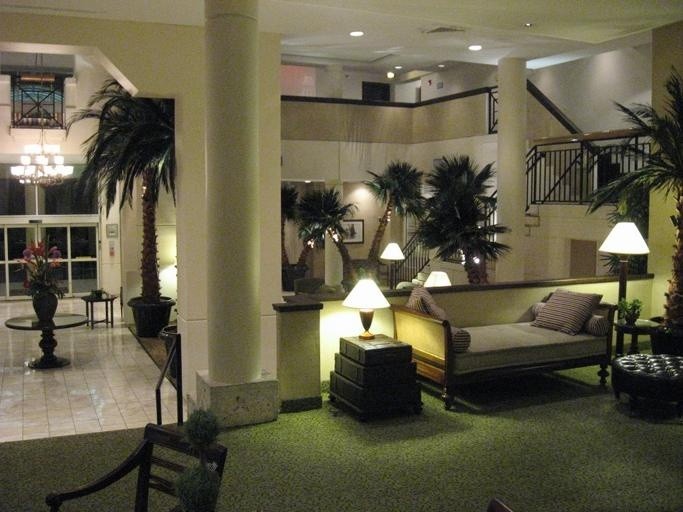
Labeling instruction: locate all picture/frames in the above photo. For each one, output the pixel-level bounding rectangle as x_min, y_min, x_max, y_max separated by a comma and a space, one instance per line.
343, 219, 364, 243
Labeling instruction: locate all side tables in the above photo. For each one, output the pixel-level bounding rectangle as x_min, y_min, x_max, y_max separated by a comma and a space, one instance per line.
613, 318, 661, 357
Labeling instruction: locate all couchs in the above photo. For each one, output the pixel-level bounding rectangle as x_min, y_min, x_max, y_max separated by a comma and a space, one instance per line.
610, 353, 683, 417
390, 292, 619, 411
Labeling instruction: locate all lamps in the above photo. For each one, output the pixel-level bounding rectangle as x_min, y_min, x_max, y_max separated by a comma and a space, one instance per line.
425, 271, 451, 287
381, 242, 406, 262
341, 280, 391, 341
598, 221, 650, 303
9, 53, 74, 187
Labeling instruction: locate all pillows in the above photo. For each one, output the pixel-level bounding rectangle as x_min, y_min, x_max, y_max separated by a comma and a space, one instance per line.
532, 287, 603, 336
406, 284, 446, 320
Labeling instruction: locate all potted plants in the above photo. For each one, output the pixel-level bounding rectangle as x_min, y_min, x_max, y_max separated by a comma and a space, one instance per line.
65, 79, 176, 338
587, 65, 683, 354
280, 185, 358, 291
618, 298, 644, 327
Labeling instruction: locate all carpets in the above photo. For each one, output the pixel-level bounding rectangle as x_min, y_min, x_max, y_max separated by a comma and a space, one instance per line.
1, 323, 682, 512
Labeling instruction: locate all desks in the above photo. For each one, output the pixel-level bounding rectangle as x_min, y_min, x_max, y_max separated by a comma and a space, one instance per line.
82, 296, 118, 329
5, 313, 88, 369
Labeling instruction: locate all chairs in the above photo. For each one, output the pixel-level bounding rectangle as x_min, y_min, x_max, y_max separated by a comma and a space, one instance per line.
45, 424, 227, 512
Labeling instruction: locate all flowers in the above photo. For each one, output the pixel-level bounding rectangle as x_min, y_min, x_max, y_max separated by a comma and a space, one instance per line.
14, 235, 69, 299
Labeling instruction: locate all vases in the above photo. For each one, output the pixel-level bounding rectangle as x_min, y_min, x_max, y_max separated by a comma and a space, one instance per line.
33, 291, 58, 325
